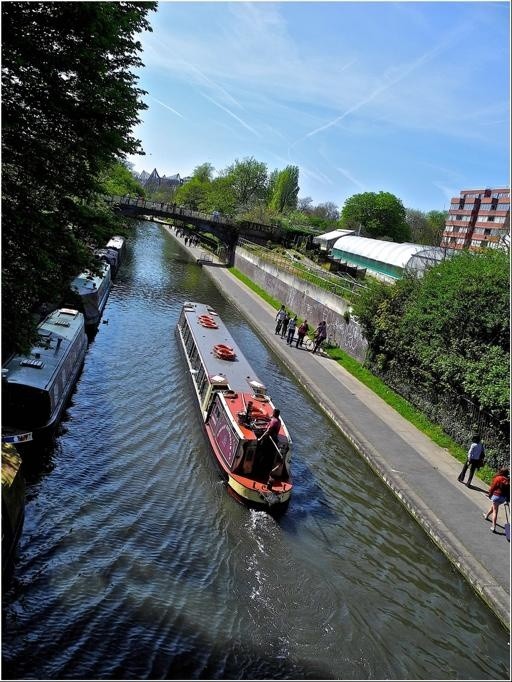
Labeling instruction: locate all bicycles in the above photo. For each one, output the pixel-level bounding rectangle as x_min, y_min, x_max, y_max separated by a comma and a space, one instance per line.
306, 333, 328, 357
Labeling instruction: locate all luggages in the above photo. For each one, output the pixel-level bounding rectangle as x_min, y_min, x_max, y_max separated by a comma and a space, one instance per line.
504, 504, 510, 541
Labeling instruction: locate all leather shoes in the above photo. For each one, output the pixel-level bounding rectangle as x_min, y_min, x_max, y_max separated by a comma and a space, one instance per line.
490, 527, 497, 534
483, 512, 488, 520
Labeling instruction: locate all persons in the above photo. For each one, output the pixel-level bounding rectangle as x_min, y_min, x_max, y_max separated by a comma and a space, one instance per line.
483, 468, 510, 532
457, 434, 485, 488
257, 408, 281, 474
170, 226, 203, 250
124, 193, 187, 216
275, 305, 327, 354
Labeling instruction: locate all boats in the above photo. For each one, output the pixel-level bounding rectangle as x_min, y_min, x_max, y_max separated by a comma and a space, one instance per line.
1, 433, 26, 585
1, 307, 88, 434
61, 260, 113, 326
176, 302, 293, 511
105, 235, 127, 267
93, 248, 120, 281
